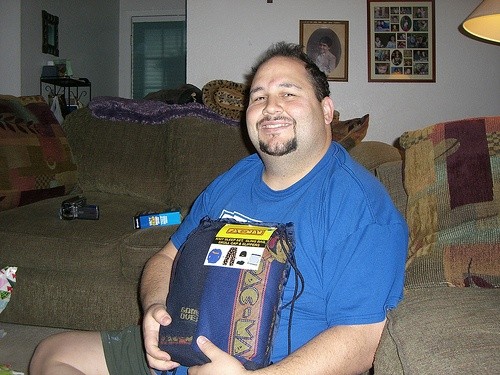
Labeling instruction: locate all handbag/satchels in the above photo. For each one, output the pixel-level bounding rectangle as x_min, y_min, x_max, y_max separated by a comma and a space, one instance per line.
157, 215, 304, 371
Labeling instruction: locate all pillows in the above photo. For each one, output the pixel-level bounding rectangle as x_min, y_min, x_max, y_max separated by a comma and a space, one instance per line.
0, 95, 78, 213
331, 114, 369, 149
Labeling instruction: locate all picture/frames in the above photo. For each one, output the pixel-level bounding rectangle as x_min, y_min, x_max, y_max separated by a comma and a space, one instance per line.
299, 19, 349, 81
366, 0, 436, 84
42, 12, 59, 57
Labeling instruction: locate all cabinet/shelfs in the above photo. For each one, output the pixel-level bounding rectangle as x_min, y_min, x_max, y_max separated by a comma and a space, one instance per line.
40, 76, 91, 112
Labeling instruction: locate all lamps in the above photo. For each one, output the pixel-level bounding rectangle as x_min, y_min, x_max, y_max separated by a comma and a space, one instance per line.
458, 0, 500, 46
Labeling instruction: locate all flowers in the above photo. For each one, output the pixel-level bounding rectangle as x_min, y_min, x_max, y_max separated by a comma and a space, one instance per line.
0, 264, 17, 375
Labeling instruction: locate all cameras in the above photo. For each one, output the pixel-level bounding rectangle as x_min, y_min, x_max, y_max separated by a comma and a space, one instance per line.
60, 195, 100, 221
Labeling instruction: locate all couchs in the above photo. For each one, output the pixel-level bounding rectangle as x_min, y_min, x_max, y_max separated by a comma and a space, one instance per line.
0, 95, 408, 332
373, 117, 500, 375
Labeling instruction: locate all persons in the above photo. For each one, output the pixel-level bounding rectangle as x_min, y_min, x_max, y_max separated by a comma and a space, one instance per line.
374, 8, 428, 75
29, 41, 409, 375
316, 36, 335, 73
